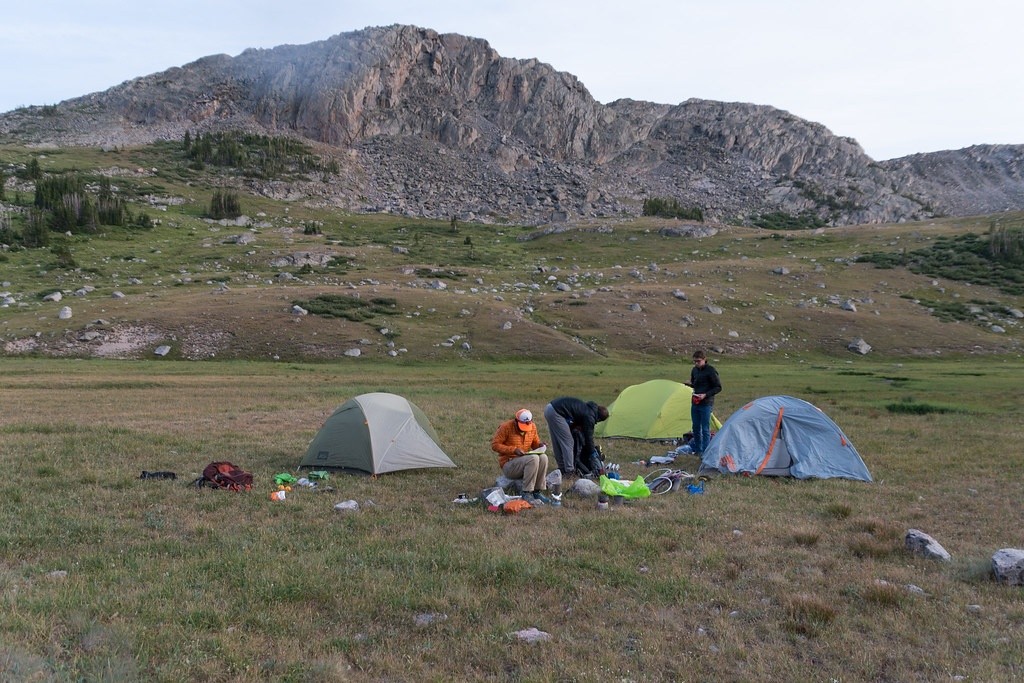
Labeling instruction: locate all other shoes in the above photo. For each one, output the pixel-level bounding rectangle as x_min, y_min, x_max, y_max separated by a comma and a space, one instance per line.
520, 492, 535, 504
559, 468, 579, 479
533, 491, 550, 503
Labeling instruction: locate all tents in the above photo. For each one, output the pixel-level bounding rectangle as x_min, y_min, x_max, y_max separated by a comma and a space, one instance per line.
300, 392, 457, 477
593, 380, 722, 440
700, 395, 872, 481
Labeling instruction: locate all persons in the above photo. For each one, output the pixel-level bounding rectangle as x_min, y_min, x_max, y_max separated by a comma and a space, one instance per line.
545, 397, 609, 475
684, 351, 722, 457
492, 409, 551, 503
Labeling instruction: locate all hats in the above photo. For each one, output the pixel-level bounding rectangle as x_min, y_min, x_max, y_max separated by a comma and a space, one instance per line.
516, 409, 532, 431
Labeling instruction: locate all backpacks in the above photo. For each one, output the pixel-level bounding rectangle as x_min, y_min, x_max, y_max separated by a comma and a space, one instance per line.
196, 461, 253, 492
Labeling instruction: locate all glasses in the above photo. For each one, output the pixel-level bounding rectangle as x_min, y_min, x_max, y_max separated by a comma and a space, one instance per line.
693, 359, 704, 363
